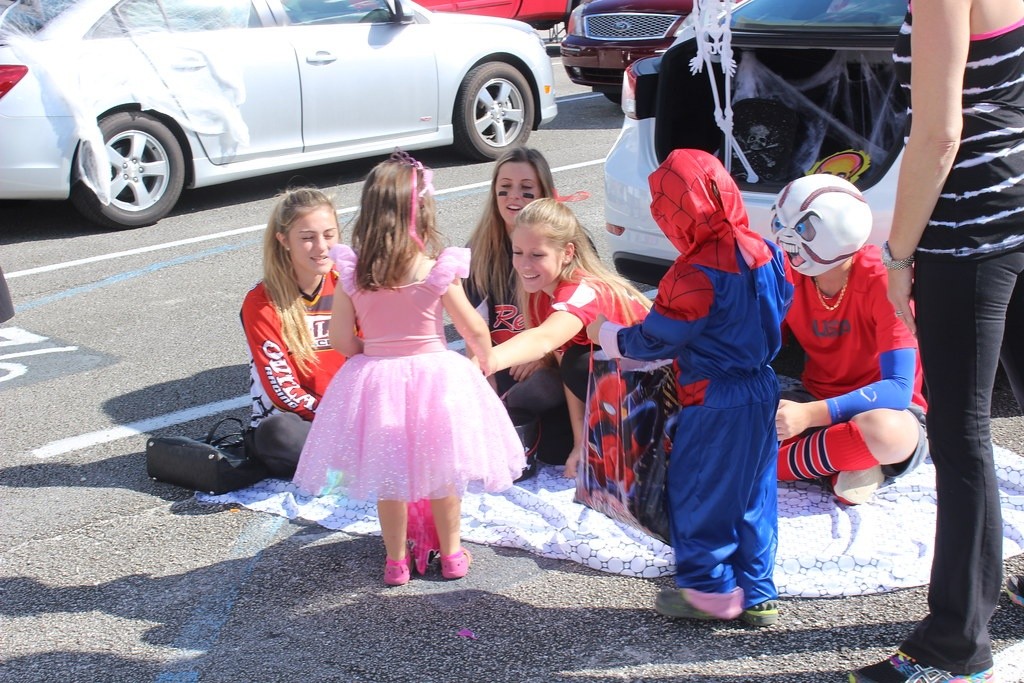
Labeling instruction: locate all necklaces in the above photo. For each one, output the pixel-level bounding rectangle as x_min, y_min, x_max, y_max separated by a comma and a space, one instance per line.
813, 255, 851, 310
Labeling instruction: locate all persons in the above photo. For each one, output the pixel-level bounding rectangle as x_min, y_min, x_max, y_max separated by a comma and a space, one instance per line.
241, 186, 364, 482
460, 145, 600, 427
294, 146, 526, 587
471, 190, 653, 480
848, 0, 1024, 683
770, 173, 932, 506
589, 148, 795, 625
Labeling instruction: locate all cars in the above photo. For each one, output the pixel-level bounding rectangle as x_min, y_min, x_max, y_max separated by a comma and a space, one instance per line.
601, 1, 916, 288
346, 0, 594, 39
0, 0, 559, 231
559, 0, 747, 105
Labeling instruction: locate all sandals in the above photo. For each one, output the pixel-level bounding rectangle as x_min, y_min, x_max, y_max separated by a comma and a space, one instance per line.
441, 547, 471, 578
384, 550, 414, 585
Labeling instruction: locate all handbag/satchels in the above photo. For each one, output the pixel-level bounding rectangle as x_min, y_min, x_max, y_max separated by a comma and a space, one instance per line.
147, 418, 271, 495
500, 392, 542, 483
573, 338, 679, 548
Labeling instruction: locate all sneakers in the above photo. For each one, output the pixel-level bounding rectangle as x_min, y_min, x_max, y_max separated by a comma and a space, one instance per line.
831, 465, 885, 506
1007, 575, 1024, 606
848, 649, 993, 683
738, 600, 779, 626
655, 589, 717, 620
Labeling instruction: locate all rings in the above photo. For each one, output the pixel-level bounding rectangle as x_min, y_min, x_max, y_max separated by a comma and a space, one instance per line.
896, 310, 903, 316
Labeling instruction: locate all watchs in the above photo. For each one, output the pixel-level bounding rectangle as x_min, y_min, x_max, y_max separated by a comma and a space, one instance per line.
881, 240, 917, 270
